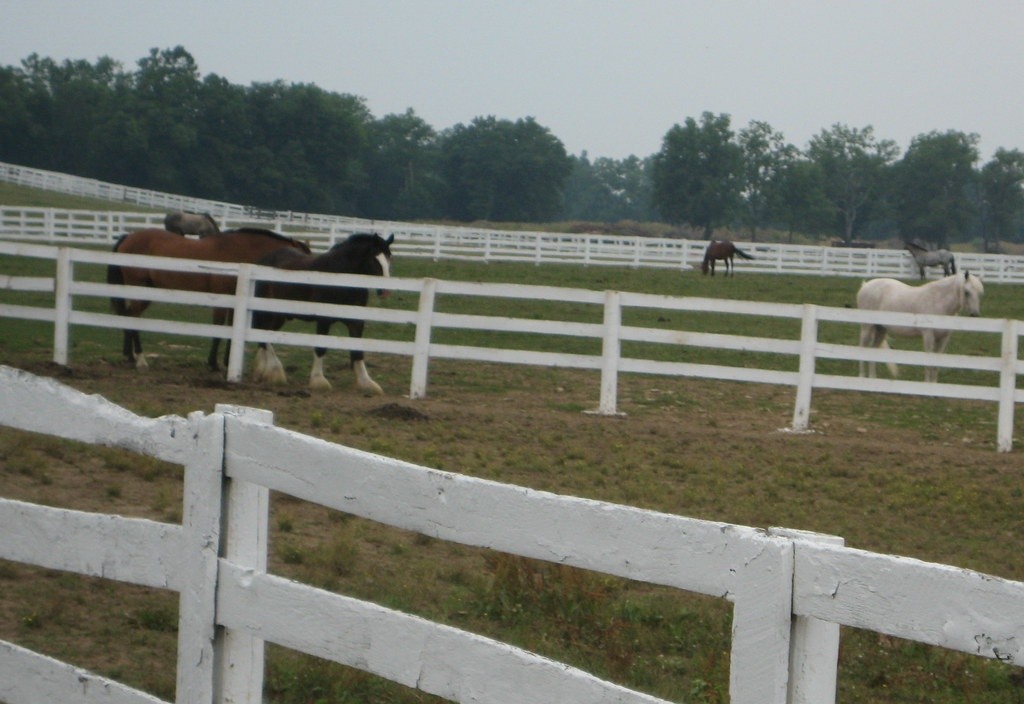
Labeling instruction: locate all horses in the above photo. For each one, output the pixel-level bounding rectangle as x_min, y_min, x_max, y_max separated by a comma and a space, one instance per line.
701, 240, 755, 277
904, 242, 957, 280
106, 210, 394, 393
857, 269, 985, 383
